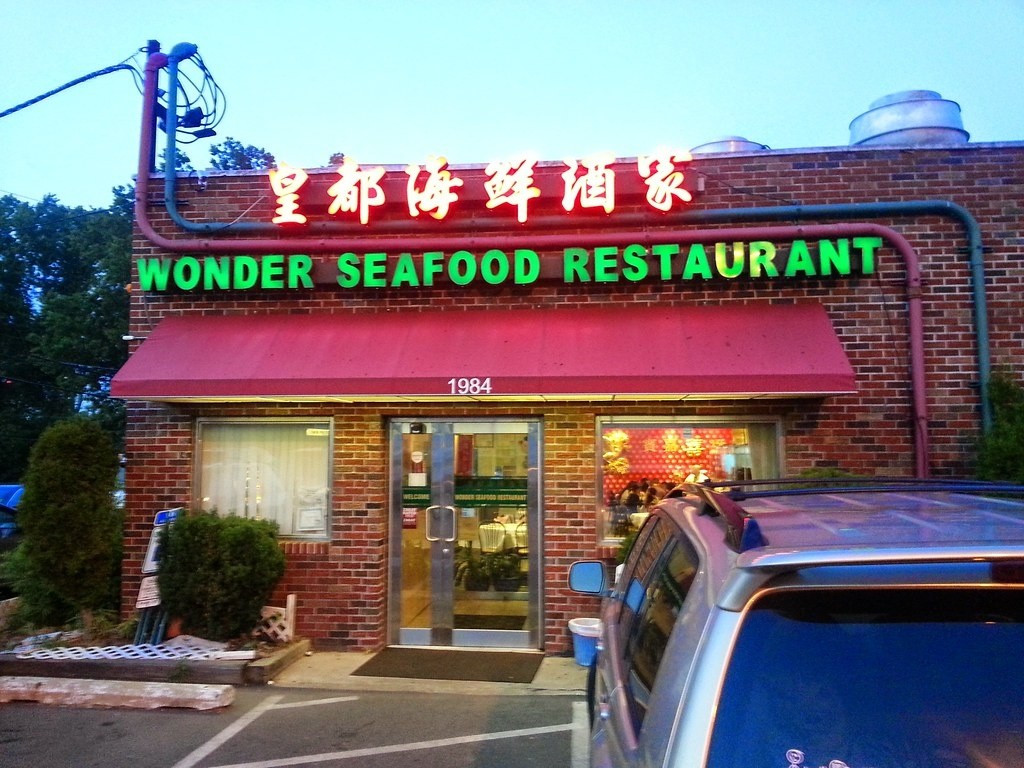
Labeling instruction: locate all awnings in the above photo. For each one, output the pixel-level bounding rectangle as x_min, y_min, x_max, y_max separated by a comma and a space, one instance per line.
106, 302, 860, 397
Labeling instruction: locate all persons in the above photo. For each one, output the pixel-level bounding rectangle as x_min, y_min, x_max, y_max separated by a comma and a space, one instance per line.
620, 465, 711, 522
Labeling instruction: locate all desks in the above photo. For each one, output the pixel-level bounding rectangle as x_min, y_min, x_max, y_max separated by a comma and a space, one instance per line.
479, 523, 529, 572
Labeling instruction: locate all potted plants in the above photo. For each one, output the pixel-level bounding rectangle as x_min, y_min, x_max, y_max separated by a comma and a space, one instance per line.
482, 547, 526, 591
452, 538, 494, 591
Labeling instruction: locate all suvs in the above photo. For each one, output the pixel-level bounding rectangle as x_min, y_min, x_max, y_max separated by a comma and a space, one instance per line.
566, 472, 1024, 768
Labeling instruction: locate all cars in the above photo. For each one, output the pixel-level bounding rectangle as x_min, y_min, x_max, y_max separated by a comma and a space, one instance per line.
0, 484, 29, 540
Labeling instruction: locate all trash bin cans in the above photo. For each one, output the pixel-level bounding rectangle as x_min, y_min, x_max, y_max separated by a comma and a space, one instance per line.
567, 617, 603, 666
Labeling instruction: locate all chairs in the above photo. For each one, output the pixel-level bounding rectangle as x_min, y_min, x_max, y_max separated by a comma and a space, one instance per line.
479, 520, 507, 555
516, 522, 529, 571
